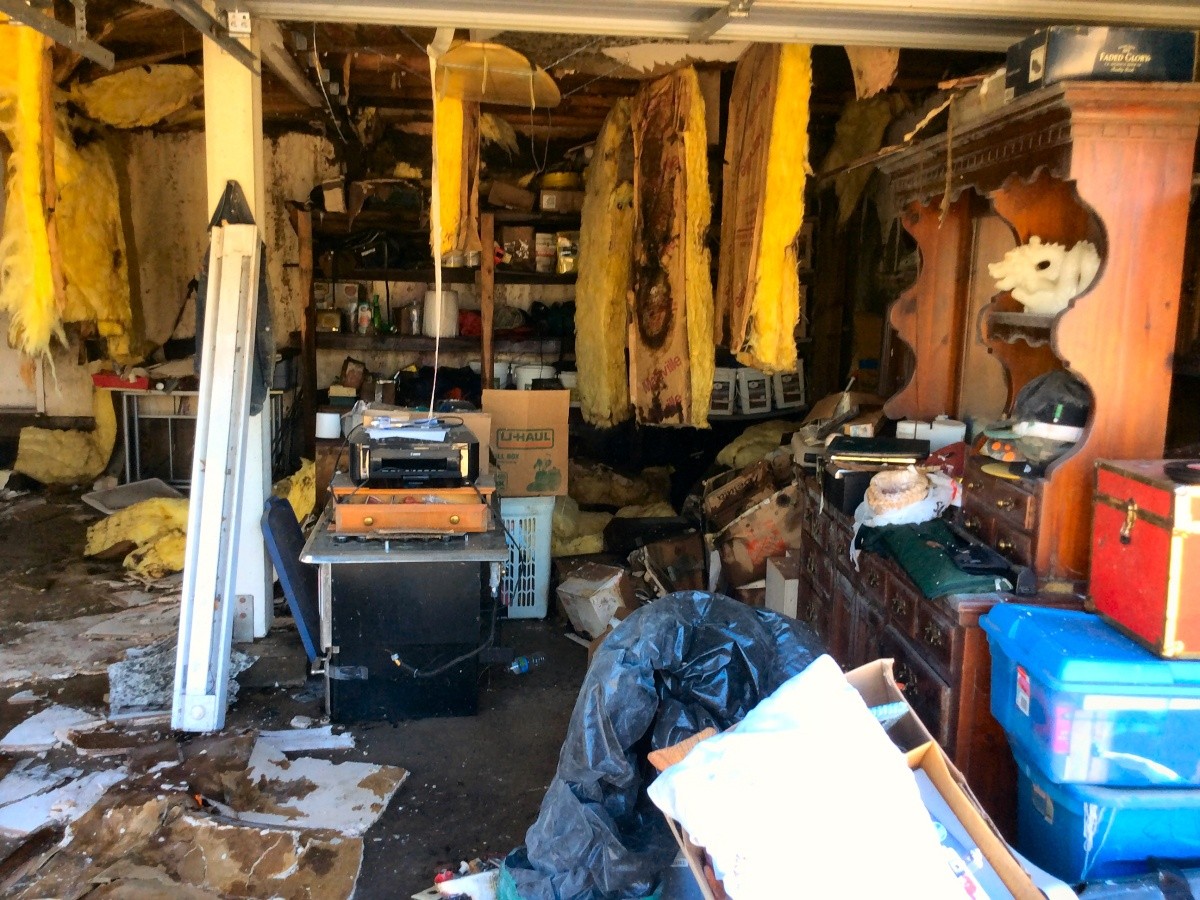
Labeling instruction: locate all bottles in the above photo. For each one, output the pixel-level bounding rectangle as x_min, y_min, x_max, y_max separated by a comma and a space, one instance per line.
372, 294, 381, 332
504, 652, 544, 674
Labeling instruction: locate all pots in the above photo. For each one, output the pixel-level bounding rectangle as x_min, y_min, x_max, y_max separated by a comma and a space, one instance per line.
392, 303, 422, 336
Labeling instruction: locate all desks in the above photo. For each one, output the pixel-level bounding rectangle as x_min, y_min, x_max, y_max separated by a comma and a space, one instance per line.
101, 385, 292, 492
298, 496, 509, 717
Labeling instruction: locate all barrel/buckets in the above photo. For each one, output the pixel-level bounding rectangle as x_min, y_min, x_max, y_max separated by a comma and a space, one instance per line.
469, 353, 581, 409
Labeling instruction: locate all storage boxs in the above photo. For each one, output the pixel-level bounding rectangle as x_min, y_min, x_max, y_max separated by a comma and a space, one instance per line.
488, 179, 536, 213
362, 380, 395, 406
482, 388, 568, 496
556, 561, 626, 640
701, 459, 770, 515
1004, 25, 1196, 92
797, 222, 813, 270
644, 657, 1049, 900
538, 190, 585, 215
91, 369, 152, 389
977, 458, 1200, 883
490, 495, 557, 620
793, 284, 807, 337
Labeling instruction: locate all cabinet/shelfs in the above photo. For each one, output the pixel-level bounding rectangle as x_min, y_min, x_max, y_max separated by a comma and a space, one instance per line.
882, 572, 1017, 850
793, 493, 839, 658
882, 76, 1200, 580
831, 527, 889, 673
300, 205, 814, 460
